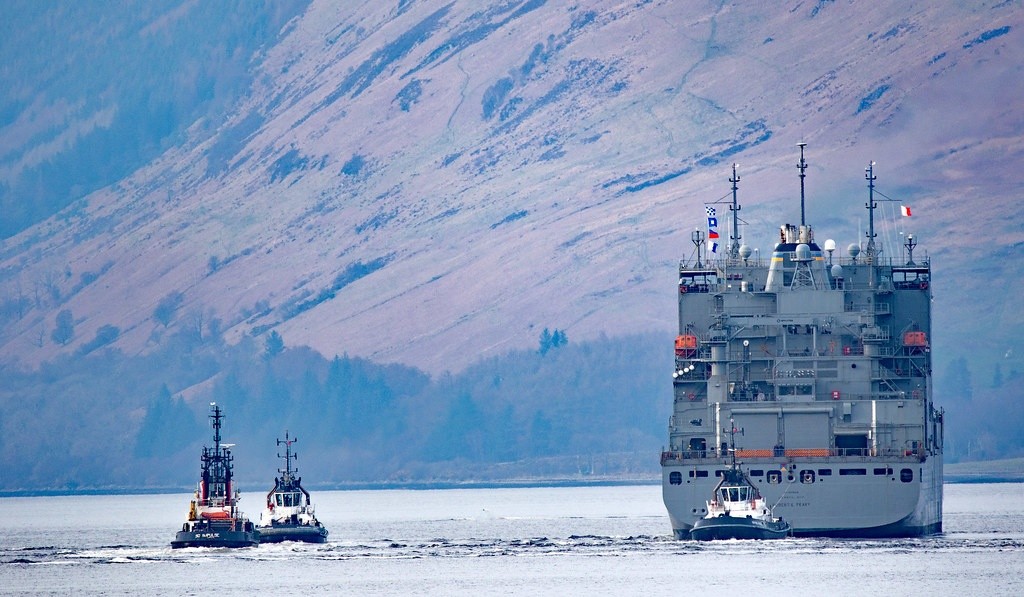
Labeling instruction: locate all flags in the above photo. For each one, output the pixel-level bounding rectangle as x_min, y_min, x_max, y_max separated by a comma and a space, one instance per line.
901, 205, 913, 217
705, 206, 720, 253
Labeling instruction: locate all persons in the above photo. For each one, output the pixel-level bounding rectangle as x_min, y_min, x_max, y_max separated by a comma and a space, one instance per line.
687, 444, 704, 458
911, 383, 923, 399
728, 381, 766, 401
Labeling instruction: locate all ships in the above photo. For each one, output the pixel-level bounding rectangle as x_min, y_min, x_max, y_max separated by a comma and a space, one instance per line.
658, 134, 948, 539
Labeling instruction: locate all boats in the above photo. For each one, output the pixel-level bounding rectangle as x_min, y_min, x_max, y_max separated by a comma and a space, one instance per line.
255, 427, 330, 546
686, 413, 793, 543
168, 403, 261, 548
